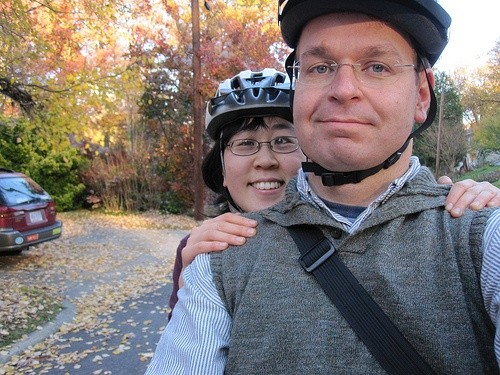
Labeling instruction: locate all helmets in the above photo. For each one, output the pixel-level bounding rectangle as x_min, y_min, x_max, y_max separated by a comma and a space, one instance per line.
277, 0, 452, 67
204, 68, 297, 140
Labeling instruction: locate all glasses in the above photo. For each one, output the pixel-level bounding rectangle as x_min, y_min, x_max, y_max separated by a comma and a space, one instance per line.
222, 136, 300, 156
287, 58, 417, 86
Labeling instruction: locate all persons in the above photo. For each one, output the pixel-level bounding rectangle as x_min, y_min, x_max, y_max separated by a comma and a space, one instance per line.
168, 68, 499, 321
145, 0, 498, 375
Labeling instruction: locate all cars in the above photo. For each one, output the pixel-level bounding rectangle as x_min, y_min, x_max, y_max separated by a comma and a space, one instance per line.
0, 172, 64, 258
